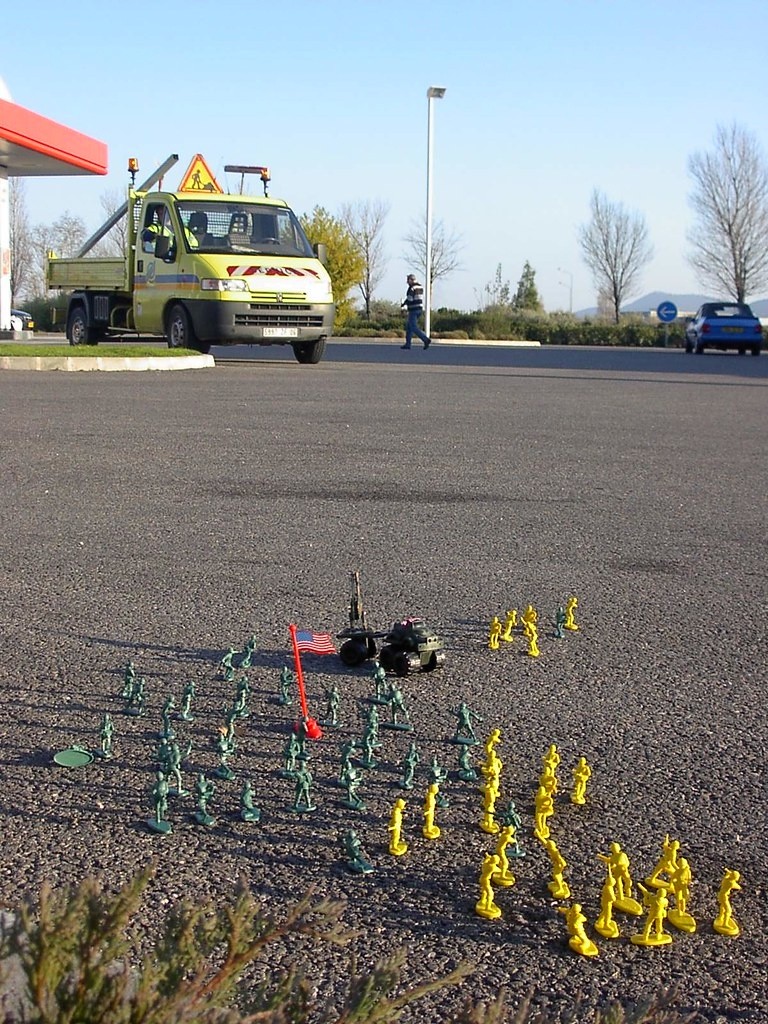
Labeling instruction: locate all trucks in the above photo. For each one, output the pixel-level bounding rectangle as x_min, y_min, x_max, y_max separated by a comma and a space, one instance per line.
42, 151, 336, 366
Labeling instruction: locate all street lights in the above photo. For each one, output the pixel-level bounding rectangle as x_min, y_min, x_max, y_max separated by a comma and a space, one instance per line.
424, 86, 448, 343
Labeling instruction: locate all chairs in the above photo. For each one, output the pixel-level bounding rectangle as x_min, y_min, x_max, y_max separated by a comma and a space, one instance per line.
186, 213, 214, 246
223, 213, 256, 246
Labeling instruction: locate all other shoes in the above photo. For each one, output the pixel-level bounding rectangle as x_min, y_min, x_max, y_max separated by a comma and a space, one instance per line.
400, 346, 411, 349
423, 338, 431, 350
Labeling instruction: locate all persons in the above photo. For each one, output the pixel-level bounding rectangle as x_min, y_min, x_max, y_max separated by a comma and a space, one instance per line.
399, 274, 432, 350
139, 204, 198, 250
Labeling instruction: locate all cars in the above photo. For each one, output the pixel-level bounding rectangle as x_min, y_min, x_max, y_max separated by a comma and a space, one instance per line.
684, 302, 764, 357
